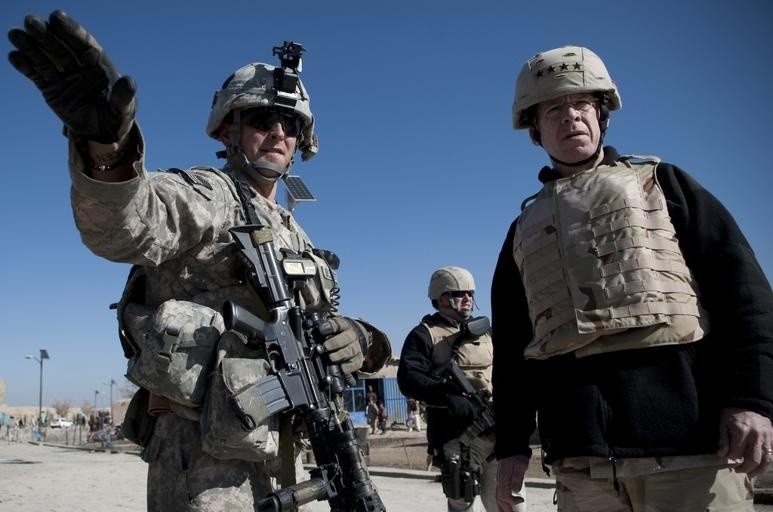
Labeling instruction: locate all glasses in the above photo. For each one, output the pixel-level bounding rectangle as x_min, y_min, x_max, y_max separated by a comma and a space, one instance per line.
242, 110, 302, 138
540, 98, 600, 120
451, 291, 475, 299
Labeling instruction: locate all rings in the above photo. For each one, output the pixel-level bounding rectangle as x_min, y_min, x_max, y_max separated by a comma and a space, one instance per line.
763, 448, 772, 455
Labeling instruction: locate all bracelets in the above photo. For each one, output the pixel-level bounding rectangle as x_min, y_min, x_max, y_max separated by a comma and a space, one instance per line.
79, 150, 129, 175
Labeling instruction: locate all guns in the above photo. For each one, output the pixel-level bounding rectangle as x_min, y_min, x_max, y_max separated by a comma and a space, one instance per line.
429, 358, 497, 449
223, 223, 388, 512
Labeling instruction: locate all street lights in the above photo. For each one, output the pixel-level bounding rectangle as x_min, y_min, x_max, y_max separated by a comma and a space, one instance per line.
89, 391, 104, 417
26, 349, 49, 441
102, 379, 117, 430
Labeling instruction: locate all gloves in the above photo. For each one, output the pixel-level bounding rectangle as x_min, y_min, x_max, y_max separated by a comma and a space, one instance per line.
442, 388, 478, 421
6, 9, 137, 146
318, 317, 373, 375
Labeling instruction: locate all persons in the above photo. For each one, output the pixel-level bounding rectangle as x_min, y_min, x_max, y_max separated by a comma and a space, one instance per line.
484, 45, 773, 512
406, 398, 420, 432
88, 408, 116, 442
377, 403, 387, 435
6, 6, 393, 509
7, 411, 49, 443
395, 265, 526, 512
365, 392, 378, 434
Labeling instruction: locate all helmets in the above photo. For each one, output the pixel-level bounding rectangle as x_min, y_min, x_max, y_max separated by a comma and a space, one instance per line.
512, 45, 623, 129
428, 266, 476, 300
205, 62, 318, 161
